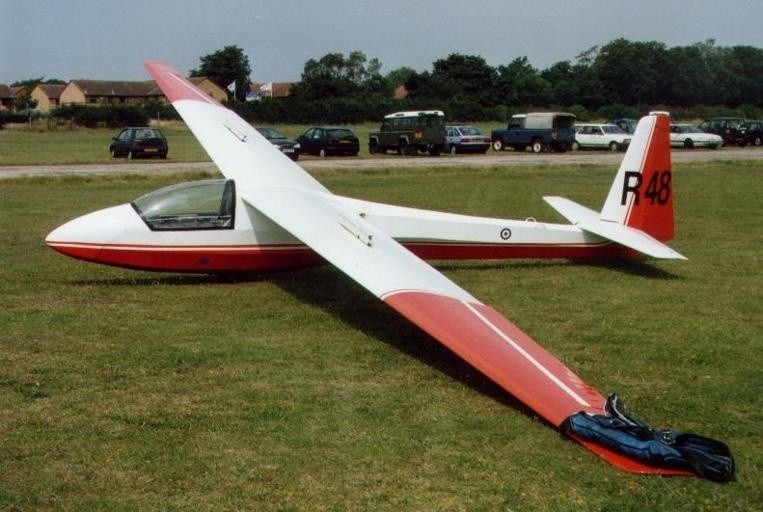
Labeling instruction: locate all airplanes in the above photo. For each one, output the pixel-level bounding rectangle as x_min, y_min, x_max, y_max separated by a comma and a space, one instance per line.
38, 59, 739, 480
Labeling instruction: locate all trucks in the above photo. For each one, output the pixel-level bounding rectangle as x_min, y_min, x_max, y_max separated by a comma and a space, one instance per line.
489, 109, 577, 153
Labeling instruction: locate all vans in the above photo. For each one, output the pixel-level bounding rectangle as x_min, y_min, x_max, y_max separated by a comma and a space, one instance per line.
695, 116, 745, 147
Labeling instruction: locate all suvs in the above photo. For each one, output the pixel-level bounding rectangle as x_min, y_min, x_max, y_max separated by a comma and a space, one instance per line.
365, 108, 447, 159
736, 120, 762, 147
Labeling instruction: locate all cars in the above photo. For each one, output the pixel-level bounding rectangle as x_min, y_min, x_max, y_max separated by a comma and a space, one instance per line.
668, 122, 724, 150
107, 125, 170, 161
572, 121, 633, 153
444, 125, 491, 155
609, 116, 637, 134
292, 124, 361, 159
252, 126, 302, 160
